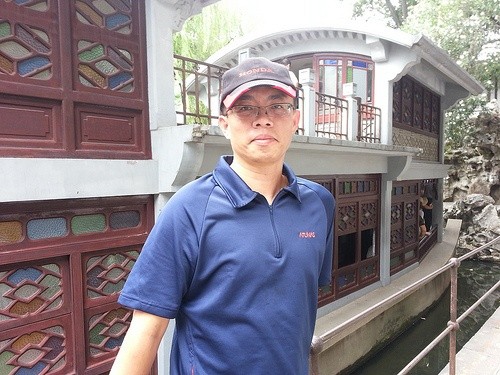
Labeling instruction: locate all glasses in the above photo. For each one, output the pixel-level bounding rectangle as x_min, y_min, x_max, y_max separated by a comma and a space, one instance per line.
224, 102, 294, 119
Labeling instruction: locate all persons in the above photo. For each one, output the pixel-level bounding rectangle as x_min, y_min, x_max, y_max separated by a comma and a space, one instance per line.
109, 57, 336, 375
418, 214, 426, 240
280, 58, 299, 91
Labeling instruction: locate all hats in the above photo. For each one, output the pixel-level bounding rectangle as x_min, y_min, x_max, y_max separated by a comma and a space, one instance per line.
221, 57, 298, 109
